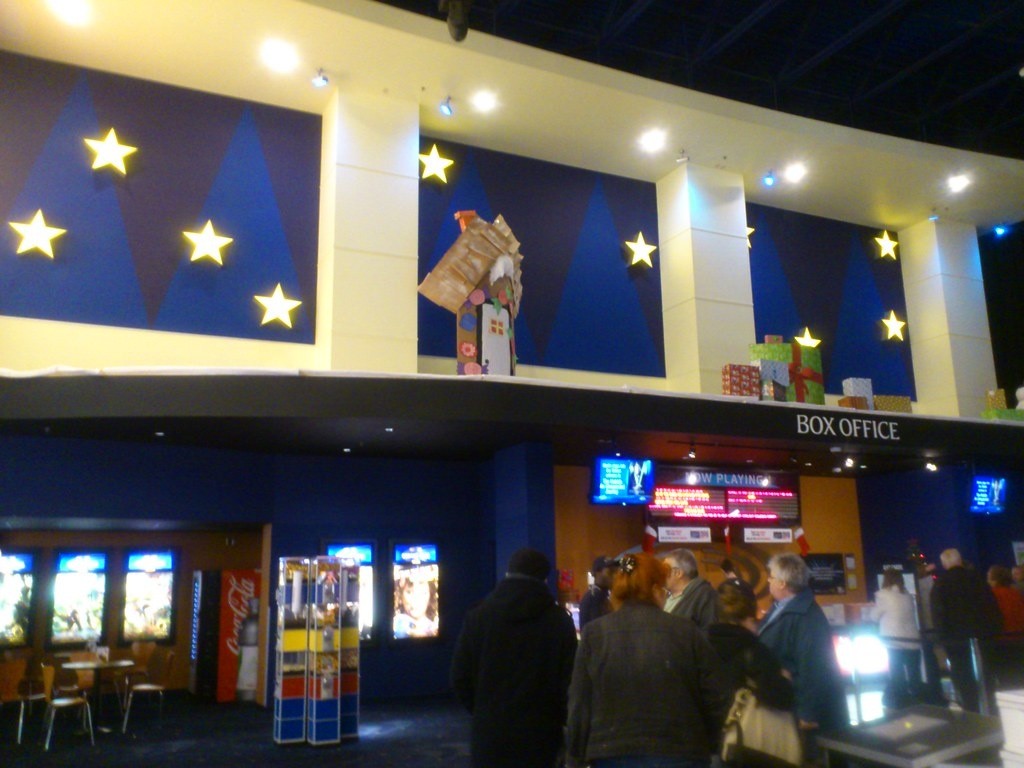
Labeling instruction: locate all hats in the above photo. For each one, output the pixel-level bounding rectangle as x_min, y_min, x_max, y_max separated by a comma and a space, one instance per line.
592, 556, 619, 576
508, 549, 551, 578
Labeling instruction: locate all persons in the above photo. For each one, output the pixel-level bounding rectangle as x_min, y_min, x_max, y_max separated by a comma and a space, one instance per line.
627, 460, 648, 495
867, 548, 1024, 720
986, 479, 1005, 507
451, 547, 578, 768
567, 548, 848, 768
389, 564, 440, 640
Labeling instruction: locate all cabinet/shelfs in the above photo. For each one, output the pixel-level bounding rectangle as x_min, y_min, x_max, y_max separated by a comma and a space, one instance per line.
274, 556, 361, 747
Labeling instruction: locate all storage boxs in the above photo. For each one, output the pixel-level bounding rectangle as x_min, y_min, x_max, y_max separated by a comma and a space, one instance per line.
722, 335, 911, 413
980, 388, 1024, 422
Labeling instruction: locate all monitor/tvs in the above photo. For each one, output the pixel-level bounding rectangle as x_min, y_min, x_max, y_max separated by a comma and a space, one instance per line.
968, 476, 1011, 515
589, 455, 656, 506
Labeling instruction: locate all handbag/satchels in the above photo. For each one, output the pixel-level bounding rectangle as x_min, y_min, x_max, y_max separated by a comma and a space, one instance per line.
723, 689, 802, 768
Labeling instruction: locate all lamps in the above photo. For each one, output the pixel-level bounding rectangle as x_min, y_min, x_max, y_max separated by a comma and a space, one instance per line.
441, 96, 452, 116
688, 446, 696, 459
764, 169, 775, 185
438, 0, 467, 41
929, 209, 938, 222
788, 450, 800, 462
310, 68, 328, 88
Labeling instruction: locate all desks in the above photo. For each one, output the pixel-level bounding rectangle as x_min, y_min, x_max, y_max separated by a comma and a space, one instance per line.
61, 659, 134, 737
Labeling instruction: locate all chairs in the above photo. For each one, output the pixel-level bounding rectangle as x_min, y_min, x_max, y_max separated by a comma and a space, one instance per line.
16, 640, 174, 751
